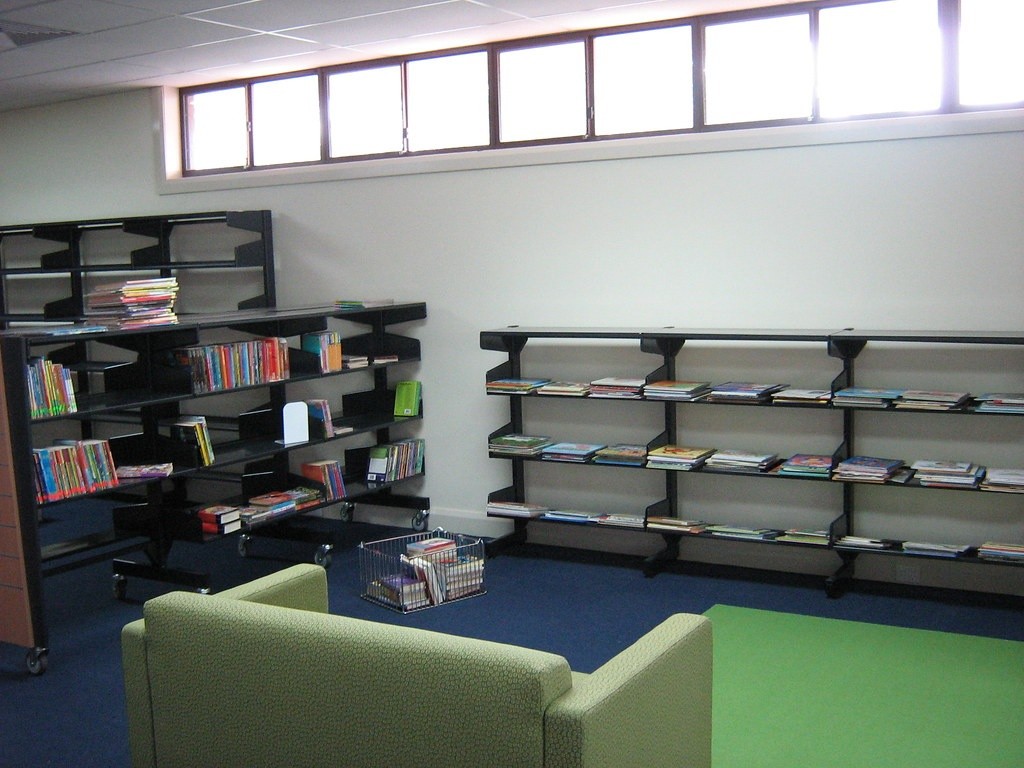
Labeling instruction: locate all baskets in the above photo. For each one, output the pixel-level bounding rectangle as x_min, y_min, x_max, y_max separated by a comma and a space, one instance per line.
358, 526, 486, 613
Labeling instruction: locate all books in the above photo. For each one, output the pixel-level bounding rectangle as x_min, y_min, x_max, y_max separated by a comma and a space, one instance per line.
300, 298, 398, 375
303, 399, 354, 440
24, 277, 216, 506
364, 380, 425, 482
366, 537, 485, 612
181, 460, 348, 536
486, 377, 1024, 569
167, 337, 290, 396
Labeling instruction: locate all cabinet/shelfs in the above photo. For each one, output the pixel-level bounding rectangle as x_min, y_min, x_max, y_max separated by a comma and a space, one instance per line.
480, 324, 1023, 600
0, 209, 428, 676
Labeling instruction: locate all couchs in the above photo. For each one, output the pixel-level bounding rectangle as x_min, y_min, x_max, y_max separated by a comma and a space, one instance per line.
123, 563, 716, 768
